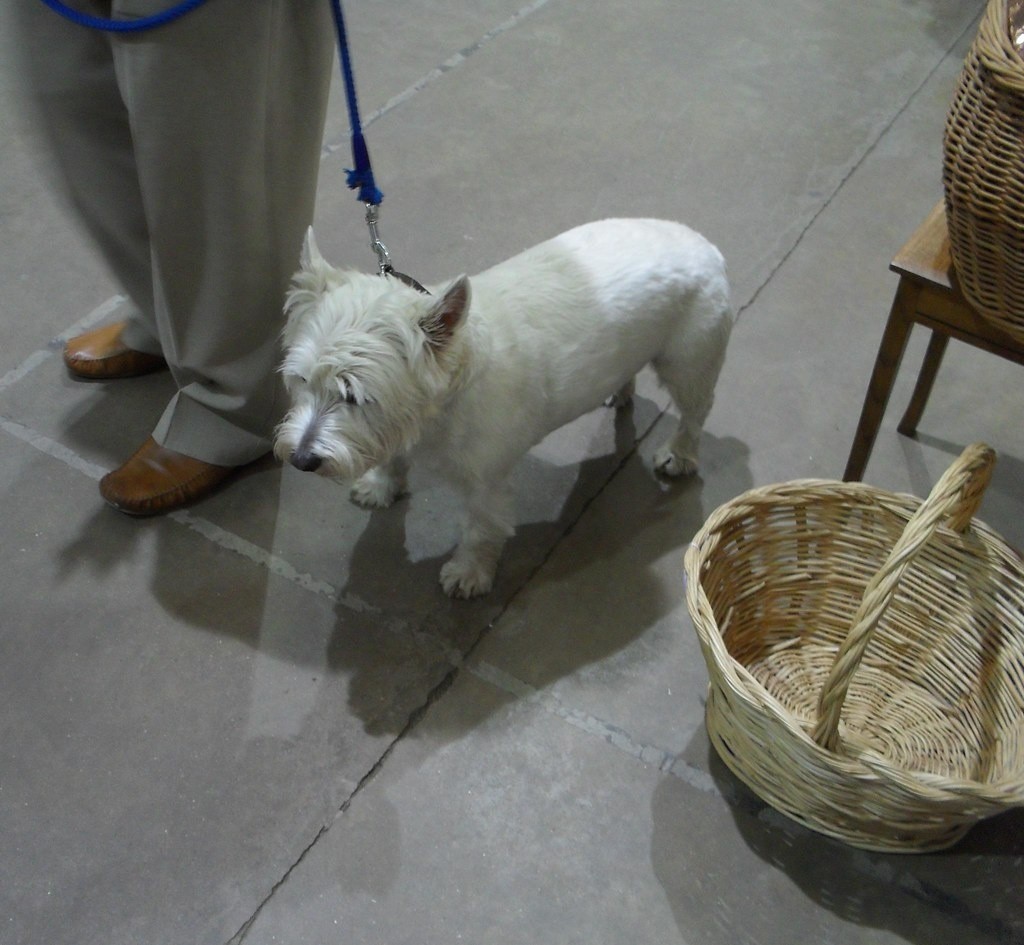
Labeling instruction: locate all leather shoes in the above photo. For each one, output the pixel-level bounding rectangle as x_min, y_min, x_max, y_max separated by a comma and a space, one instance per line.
96, 435, 279, 521
63, 316, 168, 381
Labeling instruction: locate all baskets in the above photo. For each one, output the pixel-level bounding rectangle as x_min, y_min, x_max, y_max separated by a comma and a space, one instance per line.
680, 442, 1024, 855
941, 0, 1024, 342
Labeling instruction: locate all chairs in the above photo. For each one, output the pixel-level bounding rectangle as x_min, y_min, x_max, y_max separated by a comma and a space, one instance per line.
842, 199, 1023, 490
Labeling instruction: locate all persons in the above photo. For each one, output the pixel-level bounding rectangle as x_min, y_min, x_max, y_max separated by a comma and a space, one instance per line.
0, 0, 336, 518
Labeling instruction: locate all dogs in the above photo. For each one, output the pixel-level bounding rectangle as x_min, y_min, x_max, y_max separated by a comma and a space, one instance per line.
273, 217, 735, 597
